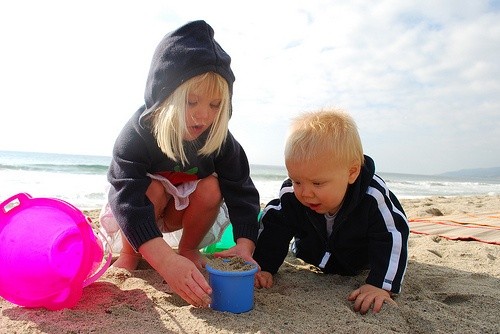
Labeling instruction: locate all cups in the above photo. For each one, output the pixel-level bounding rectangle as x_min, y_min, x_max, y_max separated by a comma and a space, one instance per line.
205, 259, 259, 314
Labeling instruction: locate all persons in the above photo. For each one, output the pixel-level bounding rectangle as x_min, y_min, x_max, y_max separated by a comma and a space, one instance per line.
99, 20, 261, 309
253, 108, 410, 315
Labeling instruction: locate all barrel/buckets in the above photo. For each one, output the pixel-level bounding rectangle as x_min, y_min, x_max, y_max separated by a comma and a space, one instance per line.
0, 193, 113, 311
